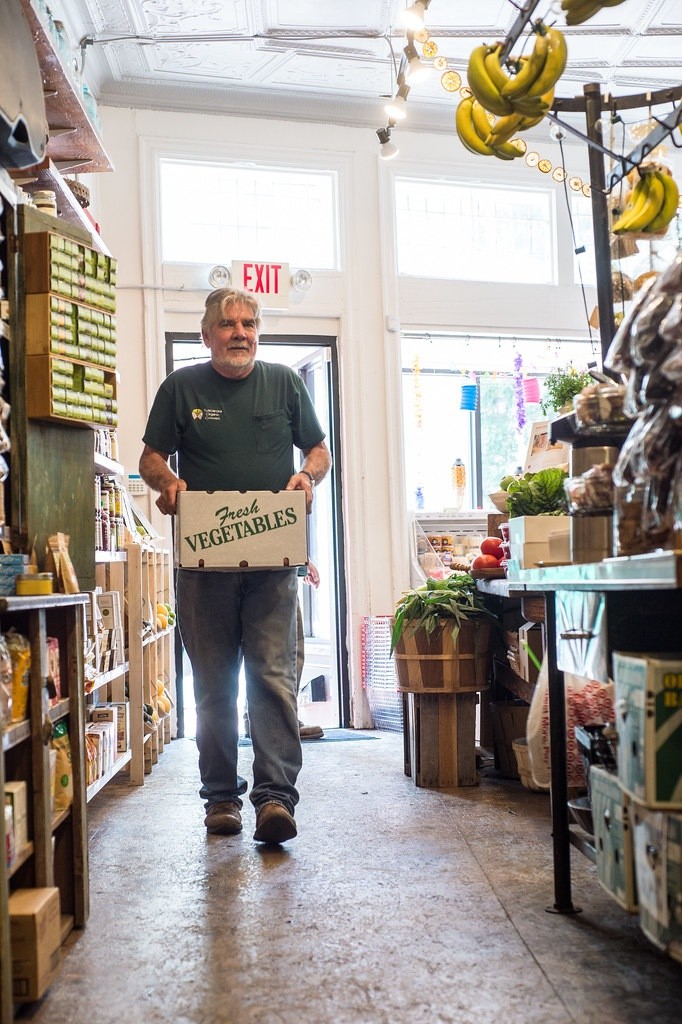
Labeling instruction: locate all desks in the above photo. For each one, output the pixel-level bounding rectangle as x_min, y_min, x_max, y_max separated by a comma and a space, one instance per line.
476, 577, 682, 914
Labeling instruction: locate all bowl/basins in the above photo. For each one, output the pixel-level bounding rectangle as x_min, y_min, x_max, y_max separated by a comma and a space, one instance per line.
565, 796, 594, 836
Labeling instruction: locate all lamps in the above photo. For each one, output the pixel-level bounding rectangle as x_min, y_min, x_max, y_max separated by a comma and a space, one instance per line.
375, 0, 432, 161
208, 260, 312, 310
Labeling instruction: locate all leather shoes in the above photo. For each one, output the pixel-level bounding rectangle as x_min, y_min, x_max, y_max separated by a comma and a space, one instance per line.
206, 804, 243, 834
300, 721, 323, 738
253, 804, 297, 843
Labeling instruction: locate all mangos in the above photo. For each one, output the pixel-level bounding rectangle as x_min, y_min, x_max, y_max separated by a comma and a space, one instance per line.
158, 603, 175, 629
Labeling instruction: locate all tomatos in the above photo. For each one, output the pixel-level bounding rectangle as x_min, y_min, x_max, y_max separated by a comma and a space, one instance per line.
470, 535, 509, 569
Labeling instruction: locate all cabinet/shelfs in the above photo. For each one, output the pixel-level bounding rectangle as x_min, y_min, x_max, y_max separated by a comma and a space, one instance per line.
0, 592, 89, 1024
0, 1, 115, 340
26, 424, 133, 805
24, 232, 120, 431
96, 543, 172, 785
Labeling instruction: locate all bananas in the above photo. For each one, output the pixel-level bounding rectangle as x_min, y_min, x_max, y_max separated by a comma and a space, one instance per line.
560, 0, 625, 24
453, 17, 566, 160
610, 163, 679, 236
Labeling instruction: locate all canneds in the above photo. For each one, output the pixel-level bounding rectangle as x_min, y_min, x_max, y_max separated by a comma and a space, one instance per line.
94, 429, 126, 552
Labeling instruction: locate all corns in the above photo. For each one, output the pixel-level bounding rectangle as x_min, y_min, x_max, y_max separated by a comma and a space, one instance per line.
394, 574, 473, 603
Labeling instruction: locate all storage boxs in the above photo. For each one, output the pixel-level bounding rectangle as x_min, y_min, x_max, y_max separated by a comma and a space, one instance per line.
588, 651, 682, 961
402, 692, 479, 788
507, 515, 573, 569
4, 591, 131, 1005
173, 491, 309, 573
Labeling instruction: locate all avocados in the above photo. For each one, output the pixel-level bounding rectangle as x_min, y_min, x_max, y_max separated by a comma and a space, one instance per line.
124, 683, 153, 727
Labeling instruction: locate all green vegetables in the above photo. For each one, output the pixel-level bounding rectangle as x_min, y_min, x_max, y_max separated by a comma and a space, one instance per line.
500, 466, 571, 518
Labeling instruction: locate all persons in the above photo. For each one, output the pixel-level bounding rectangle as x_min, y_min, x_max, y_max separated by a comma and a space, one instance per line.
244, 556, 324, 738
139, 288, 332, 839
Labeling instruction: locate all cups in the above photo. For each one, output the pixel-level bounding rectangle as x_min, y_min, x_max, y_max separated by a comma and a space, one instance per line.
599, 386, 629, 422
573, 393, 601, 429
572, 508, 612, 562
16, 573, 53, 595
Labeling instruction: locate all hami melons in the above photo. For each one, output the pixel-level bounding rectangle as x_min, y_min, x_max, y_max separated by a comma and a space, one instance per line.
156, 680, 171, 714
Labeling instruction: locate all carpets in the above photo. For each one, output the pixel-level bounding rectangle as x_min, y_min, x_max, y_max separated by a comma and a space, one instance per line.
187, 729, 382, 748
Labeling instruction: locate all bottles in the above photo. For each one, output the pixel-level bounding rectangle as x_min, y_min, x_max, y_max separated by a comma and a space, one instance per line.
32, 190, 58, 219
94, 428, 125, 552
451, 458, 465, 506
33, 0, 99, 134
415, 487, 424, 512
417, 533, 487, 581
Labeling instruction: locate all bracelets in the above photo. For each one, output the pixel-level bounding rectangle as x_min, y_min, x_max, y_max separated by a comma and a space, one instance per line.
300, 470, 315, 488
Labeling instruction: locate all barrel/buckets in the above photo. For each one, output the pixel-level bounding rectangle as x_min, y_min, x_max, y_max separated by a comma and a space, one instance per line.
512, 737, 547, 792
